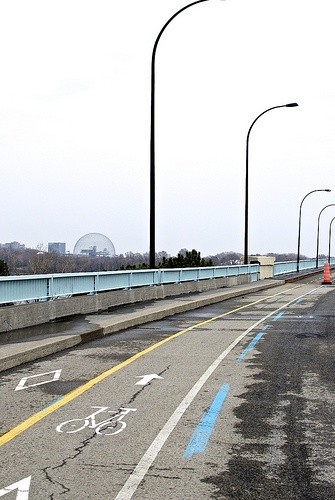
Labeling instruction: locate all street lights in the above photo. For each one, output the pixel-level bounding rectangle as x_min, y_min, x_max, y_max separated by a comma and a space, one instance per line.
149, 0, 227, 269
297, 189, 335, 273
244, 103, 300, 265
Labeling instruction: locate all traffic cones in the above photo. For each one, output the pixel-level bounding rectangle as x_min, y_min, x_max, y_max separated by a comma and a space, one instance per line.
320, 262, 332, 285
